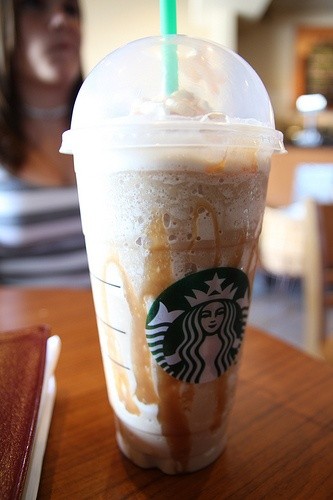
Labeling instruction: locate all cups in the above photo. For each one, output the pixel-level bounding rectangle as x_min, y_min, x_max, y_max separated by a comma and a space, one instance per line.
59, 34, 287, 476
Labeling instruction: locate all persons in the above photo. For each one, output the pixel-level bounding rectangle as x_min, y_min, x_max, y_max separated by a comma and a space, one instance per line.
0, 0, 95, 288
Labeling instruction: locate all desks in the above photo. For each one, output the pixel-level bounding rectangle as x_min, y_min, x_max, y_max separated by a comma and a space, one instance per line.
0, 287, 333, 500
